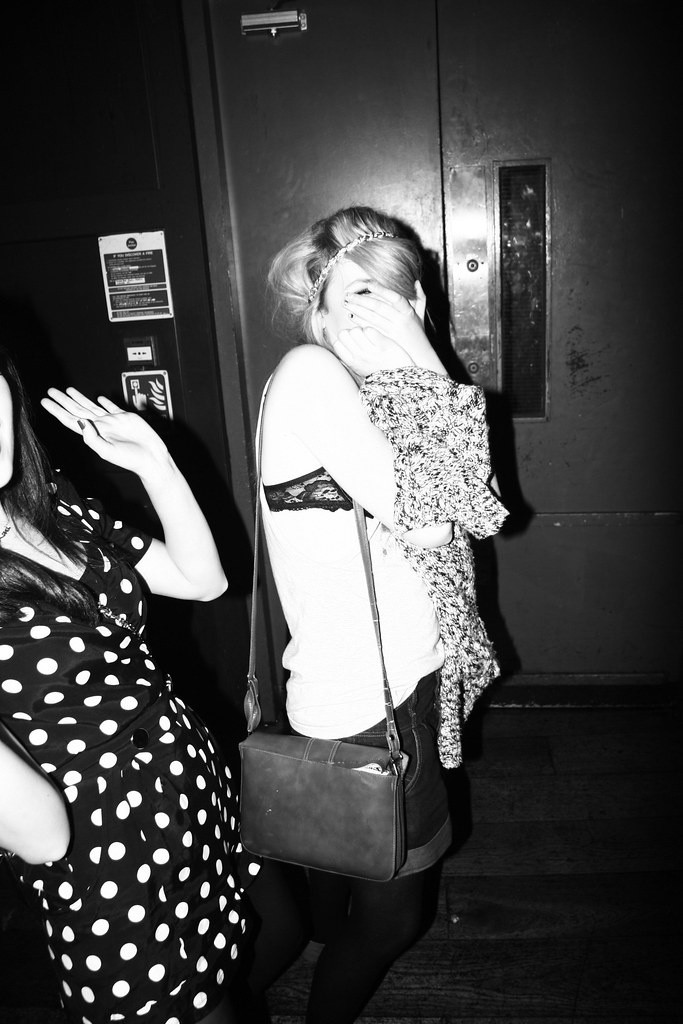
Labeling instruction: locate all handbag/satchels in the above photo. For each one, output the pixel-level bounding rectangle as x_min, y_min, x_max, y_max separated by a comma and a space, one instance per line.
234, 732, 406, 883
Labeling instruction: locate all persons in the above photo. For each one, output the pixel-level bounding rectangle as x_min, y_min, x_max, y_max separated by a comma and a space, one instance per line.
0, 312, 262, 1024
221, 207, 502, 1024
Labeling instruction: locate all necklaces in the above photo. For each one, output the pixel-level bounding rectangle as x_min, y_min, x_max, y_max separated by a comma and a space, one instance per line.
0, 490, 13, 545
